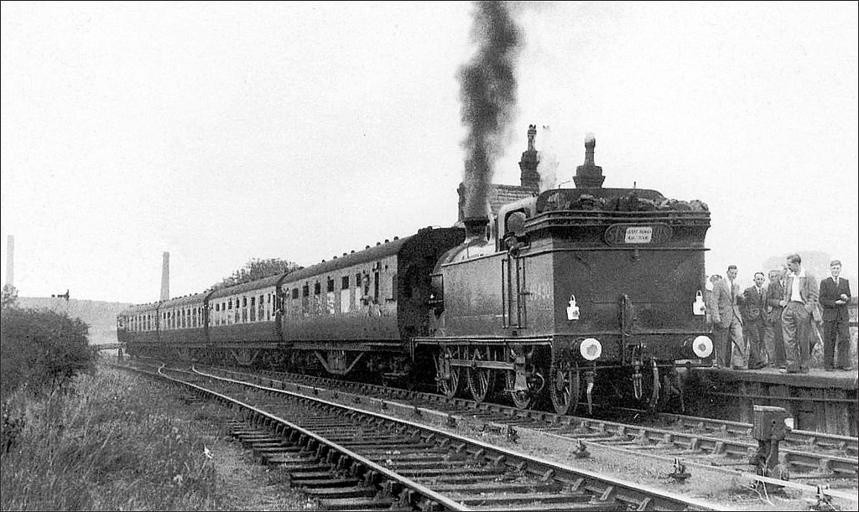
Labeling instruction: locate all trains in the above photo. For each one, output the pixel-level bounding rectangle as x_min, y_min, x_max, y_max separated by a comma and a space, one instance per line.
115, 186, 718, 418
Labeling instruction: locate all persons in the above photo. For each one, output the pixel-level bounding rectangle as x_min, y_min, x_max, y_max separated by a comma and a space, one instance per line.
703, 253, 854, 374
